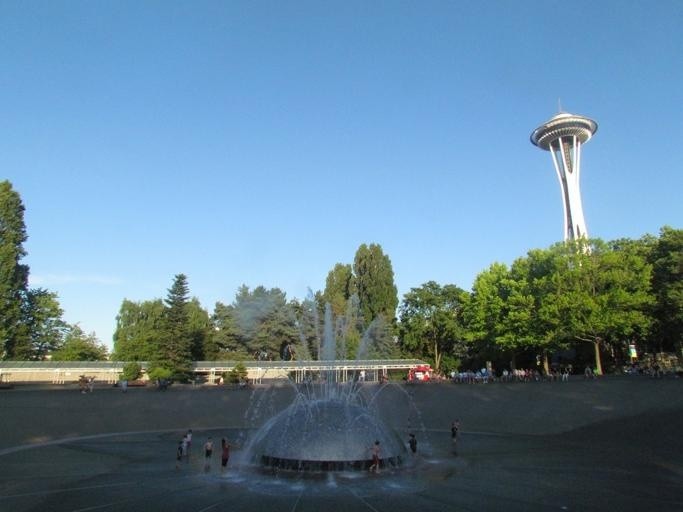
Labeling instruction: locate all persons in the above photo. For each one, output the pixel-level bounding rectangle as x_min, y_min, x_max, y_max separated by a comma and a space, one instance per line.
186, 428, 192, 450
180, 434, 188, 457
202, 436, 214, 472
174, 441, 183, 463
408, 364, 572, 385
358, 369, 366, 382
624, 359, 659, 378
450, 418, 460, 443
384, 372, 388, 385
219, 436, 240, 471
406, 431, 417, 455
585, 365, 599, 381
368, 439, 383, 473
